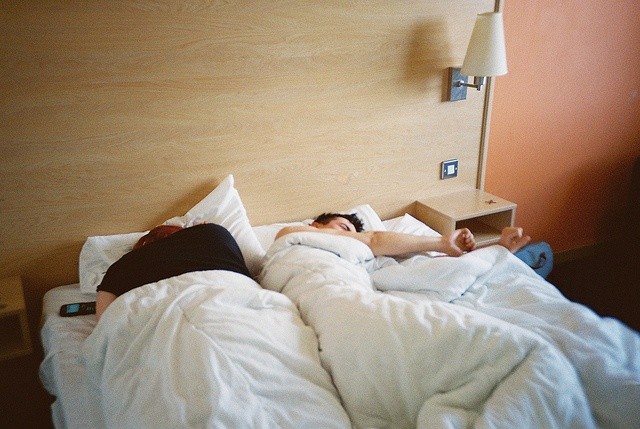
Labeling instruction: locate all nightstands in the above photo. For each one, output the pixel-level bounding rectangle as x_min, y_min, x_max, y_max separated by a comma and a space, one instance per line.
0, 273, 33, 362
416, 189, 518, 252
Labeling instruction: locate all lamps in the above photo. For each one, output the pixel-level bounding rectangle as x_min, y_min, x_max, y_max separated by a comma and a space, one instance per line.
460, 12, 509, 91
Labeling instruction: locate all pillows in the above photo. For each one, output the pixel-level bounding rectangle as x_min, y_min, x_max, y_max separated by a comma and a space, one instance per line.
251, 204, 388, 254
78, 175, 267, 294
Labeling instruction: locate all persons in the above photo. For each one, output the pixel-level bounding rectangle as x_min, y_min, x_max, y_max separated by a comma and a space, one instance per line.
275, 212, 531, 259
94, 223, 250, 322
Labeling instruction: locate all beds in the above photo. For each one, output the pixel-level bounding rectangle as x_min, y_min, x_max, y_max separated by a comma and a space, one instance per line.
40, 213, 640, 429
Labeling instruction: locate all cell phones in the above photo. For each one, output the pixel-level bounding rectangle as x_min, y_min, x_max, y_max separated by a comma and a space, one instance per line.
60, 300, 96, 317
